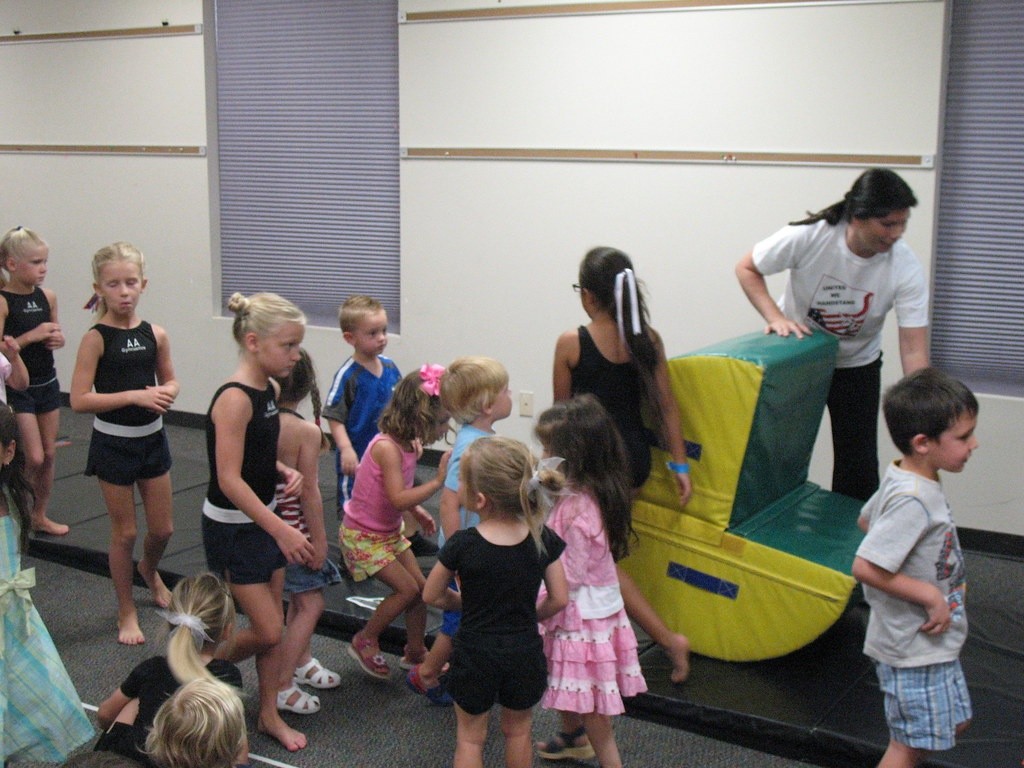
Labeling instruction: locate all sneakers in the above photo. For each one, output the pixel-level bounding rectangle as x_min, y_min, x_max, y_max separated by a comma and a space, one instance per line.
404, 664, 453, 704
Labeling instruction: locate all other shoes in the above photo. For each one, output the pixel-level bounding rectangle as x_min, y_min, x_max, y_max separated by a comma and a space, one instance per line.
406, 531, 440, 558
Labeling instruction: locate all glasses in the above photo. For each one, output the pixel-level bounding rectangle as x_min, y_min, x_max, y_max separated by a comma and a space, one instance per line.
571, 281, 587, 291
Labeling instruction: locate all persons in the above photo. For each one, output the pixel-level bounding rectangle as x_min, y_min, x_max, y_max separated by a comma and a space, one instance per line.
94, 570, 249, 768
201, 291, 342, 751
421, 437, 569, 768
0, 399, 94, 768
70, 242, 179, 645
321, 295, 440, 572
0, 226, 69, 535
852, 369, 979, 768
553, 247, 692, 683
736, 168, 931, 502
535, 396, 649, 768
337, 356, 512, 707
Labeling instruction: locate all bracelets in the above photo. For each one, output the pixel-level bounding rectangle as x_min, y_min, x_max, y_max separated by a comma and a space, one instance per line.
664, 460, 689, 473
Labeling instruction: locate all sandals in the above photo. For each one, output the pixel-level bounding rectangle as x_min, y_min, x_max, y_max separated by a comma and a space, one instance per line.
276, 657, 342, 713
537, 730, 596, 760
349, 633, 451, 681
